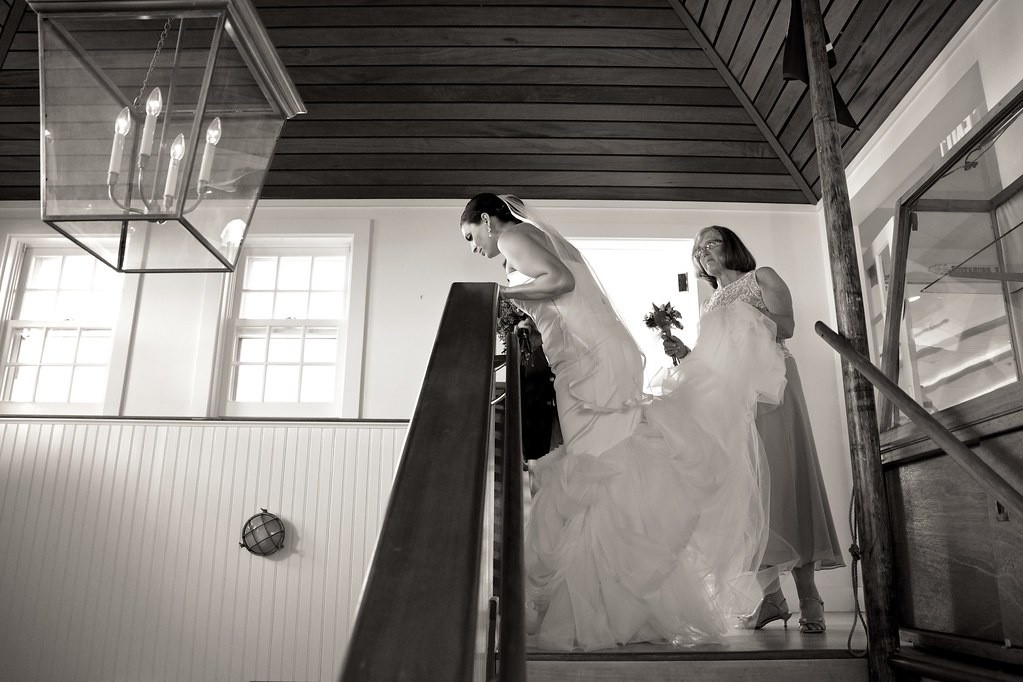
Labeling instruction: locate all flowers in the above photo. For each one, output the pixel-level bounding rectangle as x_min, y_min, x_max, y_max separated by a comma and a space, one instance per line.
495, 297, 536, 369
644, 300, 684, 368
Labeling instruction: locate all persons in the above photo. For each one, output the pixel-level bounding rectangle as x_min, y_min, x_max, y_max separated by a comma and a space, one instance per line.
688, 224, 848, 636
457, 190, 790, 657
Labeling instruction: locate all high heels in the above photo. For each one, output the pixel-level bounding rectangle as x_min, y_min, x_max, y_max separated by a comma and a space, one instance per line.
798, 597, 827, 633
734, 599, 792, 629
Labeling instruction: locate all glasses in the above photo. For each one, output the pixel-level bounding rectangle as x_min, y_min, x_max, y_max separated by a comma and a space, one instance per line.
694, 240, 723, 257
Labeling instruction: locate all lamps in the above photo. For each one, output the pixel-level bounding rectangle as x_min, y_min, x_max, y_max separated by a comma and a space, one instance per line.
29, 0, 306, 272
